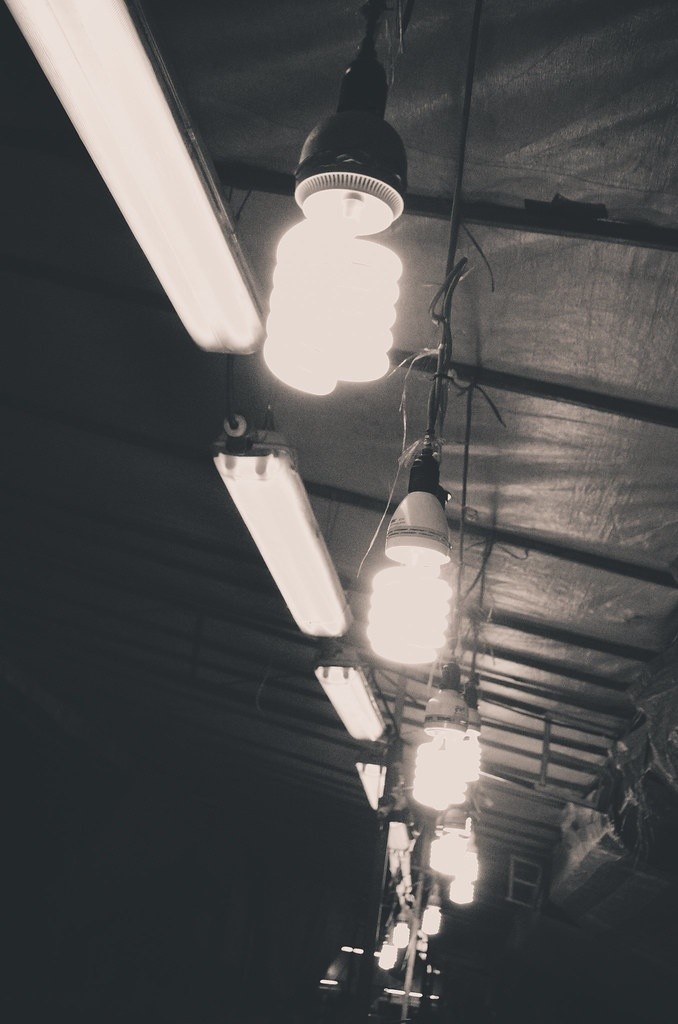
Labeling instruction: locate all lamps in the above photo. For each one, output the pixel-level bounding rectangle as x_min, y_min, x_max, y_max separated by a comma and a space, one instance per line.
5, 0, 410, 853
